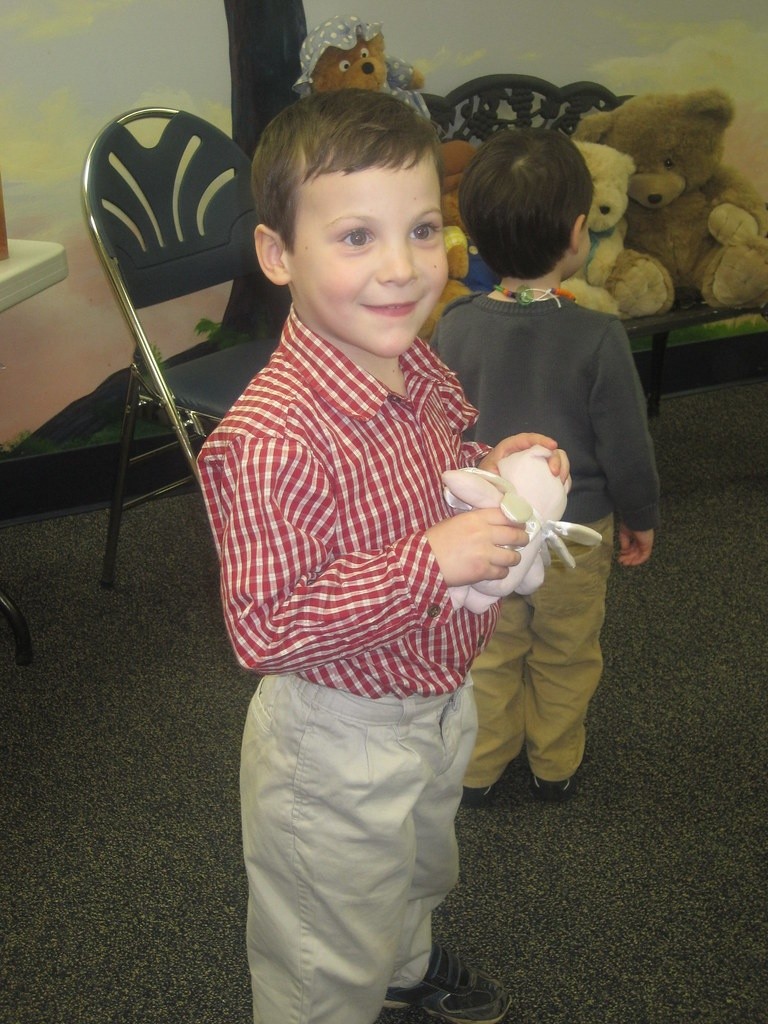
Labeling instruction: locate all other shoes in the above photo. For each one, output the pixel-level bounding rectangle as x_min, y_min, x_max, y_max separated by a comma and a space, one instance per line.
531, 774, 577, 803
461, 784, 498, 808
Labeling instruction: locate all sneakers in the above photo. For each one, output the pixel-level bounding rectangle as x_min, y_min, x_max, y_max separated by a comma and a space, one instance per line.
383, 942, 513, 1024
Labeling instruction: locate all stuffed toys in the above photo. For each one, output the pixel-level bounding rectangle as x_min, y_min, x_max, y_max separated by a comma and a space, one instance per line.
440, 444, 604, 614
294, 14, 768, 342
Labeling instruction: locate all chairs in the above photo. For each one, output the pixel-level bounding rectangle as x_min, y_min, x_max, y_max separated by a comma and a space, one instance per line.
79, 106, 292, 585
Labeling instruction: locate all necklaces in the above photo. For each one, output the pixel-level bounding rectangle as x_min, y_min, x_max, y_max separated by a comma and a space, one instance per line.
493, 283, 576, 305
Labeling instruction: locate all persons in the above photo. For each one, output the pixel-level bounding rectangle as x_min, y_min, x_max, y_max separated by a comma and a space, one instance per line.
428, 126, 659, 805
197, 88, 571, 1023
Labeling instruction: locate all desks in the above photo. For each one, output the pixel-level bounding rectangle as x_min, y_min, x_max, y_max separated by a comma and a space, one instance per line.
622, 287, 768, 418
0, 240, 68, 667
